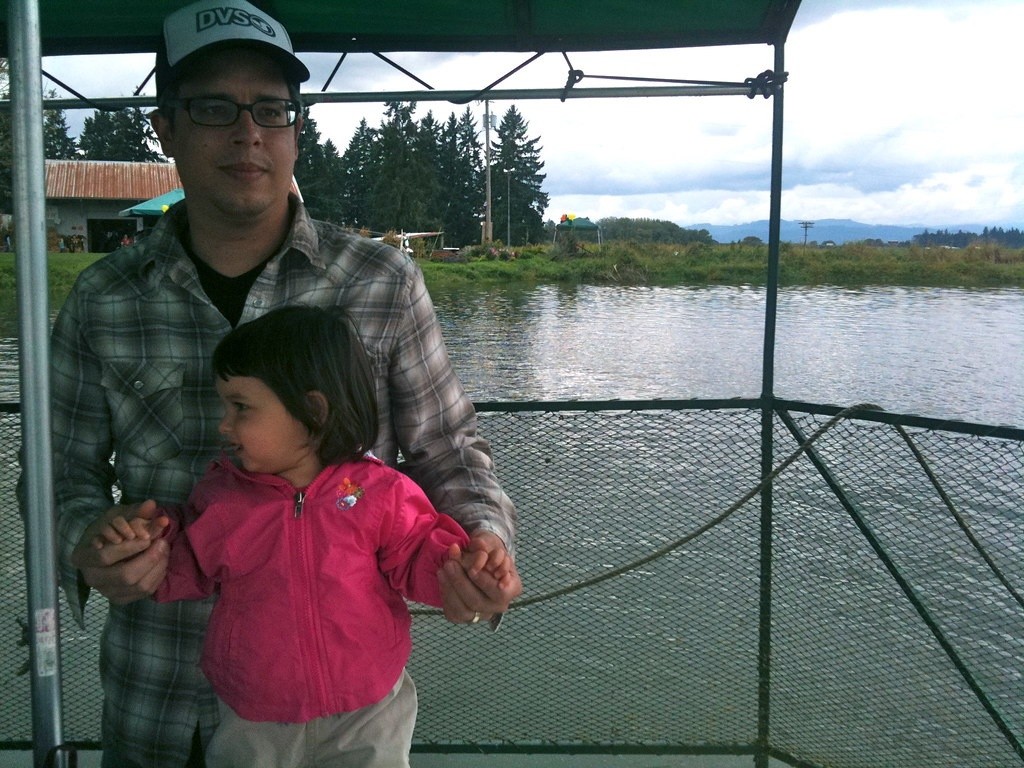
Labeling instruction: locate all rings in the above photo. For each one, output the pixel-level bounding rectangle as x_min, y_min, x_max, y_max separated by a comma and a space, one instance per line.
468, 610, 481, 625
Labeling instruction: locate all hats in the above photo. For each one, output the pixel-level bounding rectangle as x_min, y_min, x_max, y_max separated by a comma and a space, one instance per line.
156, 0, 310, 94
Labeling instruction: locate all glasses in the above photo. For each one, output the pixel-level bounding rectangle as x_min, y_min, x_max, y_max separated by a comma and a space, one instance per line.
163, 96, 301, 128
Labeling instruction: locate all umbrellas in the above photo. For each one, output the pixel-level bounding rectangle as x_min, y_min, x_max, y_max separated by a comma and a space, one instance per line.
118, 187, 186, 217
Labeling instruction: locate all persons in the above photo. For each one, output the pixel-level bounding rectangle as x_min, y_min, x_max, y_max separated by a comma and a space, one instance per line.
4, 226, 134, 252
90, 301, 511, 768
14, 0, 523, 768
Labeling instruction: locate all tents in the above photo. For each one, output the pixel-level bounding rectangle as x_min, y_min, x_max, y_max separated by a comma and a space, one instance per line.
552, 213, 603, 245
